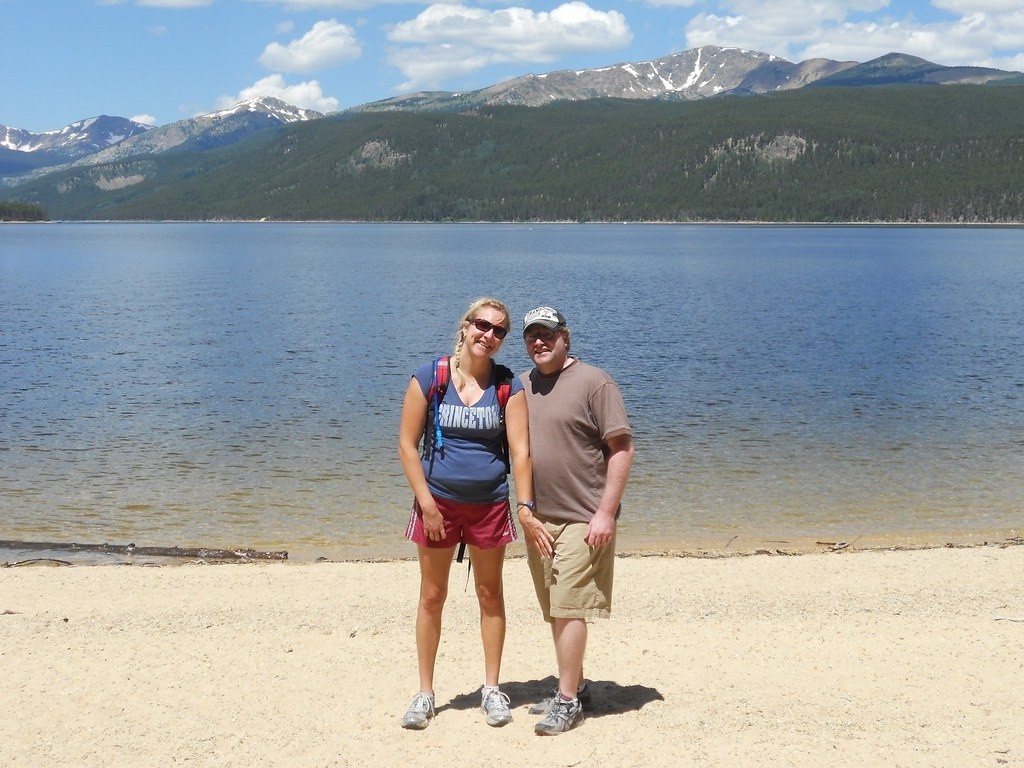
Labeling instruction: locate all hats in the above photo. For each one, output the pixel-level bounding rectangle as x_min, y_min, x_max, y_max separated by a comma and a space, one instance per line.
522, 306, 566, 338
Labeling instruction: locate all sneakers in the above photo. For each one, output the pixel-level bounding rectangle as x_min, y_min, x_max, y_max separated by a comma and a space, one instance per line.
401, 690, 435, 730
535, 687, 584, 736
481, 684, 512, 727
529, 682, 593, 714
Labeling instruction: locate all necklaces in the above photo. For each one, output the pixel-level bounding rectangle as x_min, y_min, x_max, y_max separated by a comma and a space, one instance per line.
463, 375, 480, 391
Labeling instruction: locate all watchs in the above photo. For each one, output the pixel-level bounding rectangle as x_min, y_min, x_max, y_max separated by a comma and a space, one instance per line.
516, 501, 535, 510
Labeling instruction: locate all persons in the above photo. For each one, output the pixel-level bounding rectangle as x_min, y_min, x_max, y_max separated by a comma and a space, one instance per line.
398, 298, 554, 729
518, 308, 633, 736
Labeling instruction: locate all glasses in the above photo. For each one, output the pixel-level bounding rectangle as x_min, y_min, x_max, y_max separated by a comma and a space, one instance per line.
466, 318, 507, 339
524, 327, 564, 343
